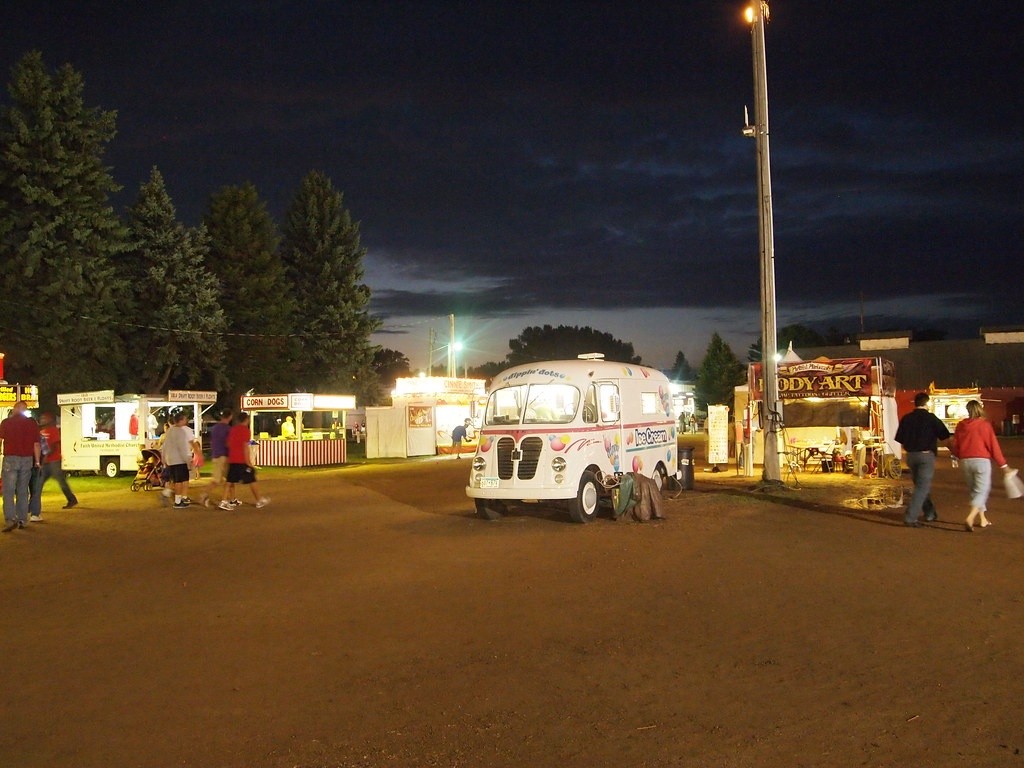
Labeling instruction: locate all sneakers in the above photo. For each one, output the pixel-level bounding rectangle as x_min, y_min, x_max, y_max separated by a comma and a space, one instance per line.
229, 498, 243, 506
173, 500, 188, 508
217, 500, 234, 510
201, 490, 211, 507
182, 497, 195, 504
256, 496, 270, 508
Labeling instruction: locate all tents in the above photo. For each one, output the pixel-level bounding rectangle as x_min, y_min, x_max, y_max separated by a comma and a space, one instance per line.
734, 357, 901, 478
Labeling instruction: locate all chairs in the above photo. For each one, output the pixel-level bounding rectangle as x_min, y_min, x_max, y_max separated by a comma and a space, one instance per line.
811, 441, 835, 473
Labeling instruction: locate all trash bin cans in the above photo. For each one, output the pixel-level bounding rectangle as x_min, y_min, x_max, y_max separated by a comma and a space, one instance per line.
667, 446, 696, 490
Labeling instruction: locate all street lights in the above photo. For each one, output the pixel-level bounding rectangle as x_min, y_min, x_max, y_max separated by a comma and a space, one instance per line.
740, 0, 782, 482
447, 343, 462, 378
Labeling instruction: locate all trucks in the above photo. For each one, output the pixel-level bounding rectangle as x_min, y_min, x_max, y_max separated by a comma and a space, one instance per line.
57, 389, 217, 478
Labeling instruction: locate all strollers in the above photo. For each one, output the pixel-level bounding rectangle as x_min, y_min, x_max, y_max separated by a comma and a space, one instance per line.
130, 447, 165, 492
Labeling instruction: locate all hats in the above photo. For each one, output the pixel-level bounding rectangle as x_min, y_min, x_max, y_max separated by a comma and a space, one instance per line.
286, 416, 293, 421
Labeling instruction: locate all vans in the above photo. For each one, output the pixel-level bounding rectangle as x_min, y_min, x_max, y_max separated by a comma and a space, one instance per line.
464, 352, 682, 524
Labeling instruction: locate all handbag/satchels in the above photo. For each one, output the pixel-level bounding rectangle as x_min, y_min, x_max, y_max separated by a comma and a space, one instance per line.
1003, 469, 1024, 499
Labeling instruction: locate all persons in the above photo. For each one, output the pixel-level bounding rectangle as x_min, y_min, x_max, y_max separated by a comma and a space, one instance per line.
353, 422, 359, 432
678, 412, 685, 434
201, 408, 271, 511
449, 425, 470, 460
360, 420, 365, 432
688, 414, 696, 434
281, 416, 295, 438
154, 412, 205, 508
895, 393, 951, 526
955, 401, 1011, 531
0, 401, 78, 533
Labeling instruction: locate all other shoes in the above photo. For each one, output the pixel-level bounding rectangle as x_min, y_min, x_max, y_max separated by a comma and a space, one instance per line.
27, 504, 31, 513
926, 512, 937, 520
2, 517, 18, 531
904, 520, 924, 527
964, 521, 975, 532
30, 515, 44, 522
195, 476, 201, 480
980, 520, 992, 528
19, 520, 24, 529
62, 499, 78, 508
162, 488, 173, 497
